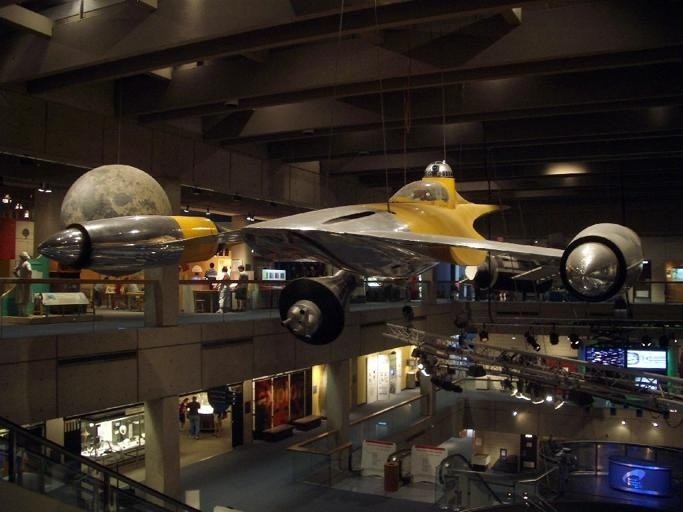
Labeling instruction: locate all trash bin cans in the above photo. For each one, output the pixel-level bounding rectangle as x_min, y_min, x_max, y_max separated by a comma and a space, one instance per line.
384, 462, 400, 492
406, 372, 416, 389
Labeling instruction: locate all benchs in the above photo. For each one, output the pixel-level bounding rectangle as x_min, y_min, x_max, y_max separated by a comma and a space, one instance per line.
259, 421, 295, 443
293, 412, 324, 433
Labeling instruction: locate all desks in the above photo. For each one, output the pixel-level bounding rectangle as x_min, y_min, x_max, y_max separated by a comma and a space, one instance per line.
80, 277, 235, 313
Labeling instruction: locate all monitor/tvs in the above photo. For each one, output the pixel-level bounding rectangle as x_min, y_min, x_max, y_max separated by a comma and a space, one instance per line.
626, 348, 667, 372
584, 346, 626, 368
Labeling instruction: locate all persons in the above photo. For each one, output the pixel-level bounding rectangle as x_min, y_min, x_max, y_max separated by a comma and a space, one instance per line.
554, 446, 573, 492
178, 398, 189, 431
211, 409, 223, 438
184, 397, 200, 440
380, 277, 393, 301
90, 262, 262, 314
11, 251, 32, 316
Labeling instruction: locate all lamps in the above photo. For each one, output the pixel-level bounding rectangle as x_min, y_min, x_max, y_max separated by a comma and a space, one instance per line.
190, 263, 204, 277
